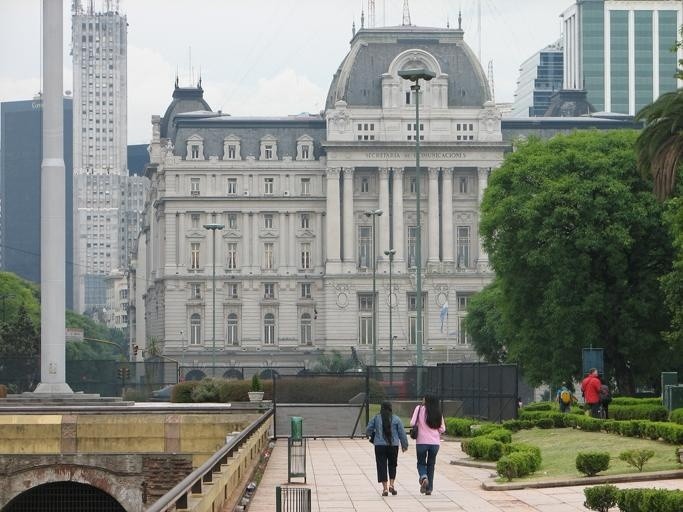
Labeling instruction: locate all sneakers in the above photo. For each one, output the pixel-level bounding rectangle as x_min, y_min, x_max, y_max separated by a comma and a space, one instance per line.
420, 478, 431, 495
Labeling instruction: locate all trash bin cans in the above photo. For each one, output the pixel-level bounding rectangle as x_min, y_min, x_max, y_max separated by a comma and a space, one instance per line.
291, 417, 303, 446
276, 487, 311, 512
288, 437, 306, 483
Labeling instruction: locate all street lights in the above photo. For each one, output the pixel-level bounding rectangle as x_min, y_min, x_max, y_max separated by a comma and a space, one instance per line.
200, 222, 226, 375
382, 247, 397, 386
359, 207, 385, 375
397, 67, 437, 399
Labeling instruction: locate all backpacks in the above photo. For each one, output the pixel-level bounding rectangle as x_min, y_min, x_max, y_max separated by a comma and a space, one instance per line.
561, 390, 571, 404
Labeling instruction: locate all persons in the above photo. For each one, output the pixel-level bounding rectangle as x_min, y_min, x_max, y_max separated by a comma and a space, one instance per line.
364, 400, 409, 496
409, 393, 446, 496
555, 382, 578, 413
581, 368, 610, 420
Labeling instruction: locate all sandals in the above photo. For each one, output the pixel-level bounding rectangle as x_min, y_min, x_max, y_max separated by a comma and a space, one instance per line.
382, 485, 397, 496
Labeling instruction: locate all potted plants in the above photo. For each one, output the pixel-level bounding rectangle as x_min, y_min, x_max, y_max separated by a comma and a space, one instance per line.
246, 374, 265, 402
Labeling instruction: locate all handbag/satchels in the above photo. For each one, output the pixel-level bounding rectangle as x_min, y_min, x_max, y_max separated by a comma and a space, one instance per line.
410, 426, 418, 439
